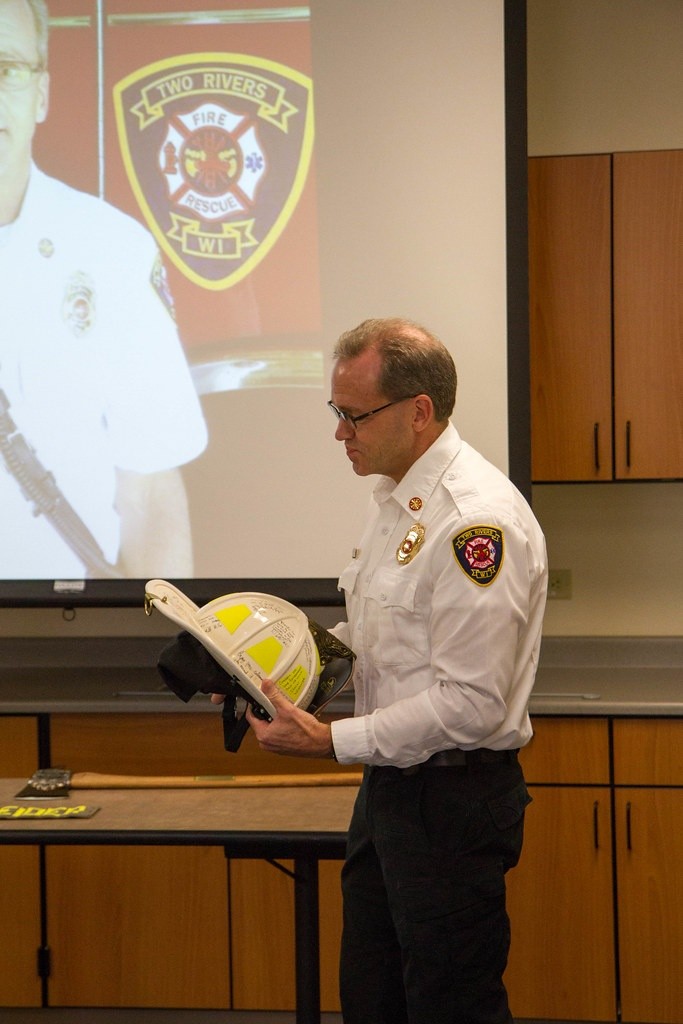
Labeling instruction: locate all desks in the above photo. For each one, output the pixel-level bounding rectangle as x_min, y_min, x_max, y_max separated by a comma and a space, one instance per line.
0, 778, 361, 1024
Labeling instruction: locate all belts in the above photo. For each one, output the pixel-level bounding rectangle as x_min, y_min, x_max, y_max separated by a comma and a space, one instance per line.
418, 748, 517, 767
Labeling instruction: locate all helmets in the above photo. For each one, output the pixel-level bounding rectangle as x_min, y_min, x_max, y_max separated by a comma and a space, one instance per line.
145, 578, 320, 723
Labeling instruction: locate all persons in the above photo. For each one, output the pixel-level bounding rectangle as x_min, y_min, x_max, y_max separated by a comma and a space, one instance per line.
212, 316, 549, 1023
1, 1, 210, 579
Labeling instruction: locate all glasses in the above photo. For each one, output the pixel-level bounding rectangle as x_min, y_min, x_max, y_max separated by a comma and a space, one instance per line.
327, 395, 415, 431
0, 61, 43, 91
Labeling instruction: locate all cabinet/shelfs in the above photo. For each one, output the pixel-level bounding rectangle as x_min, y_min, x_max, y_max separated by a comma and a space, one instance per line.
1, 150, 683, 1024
